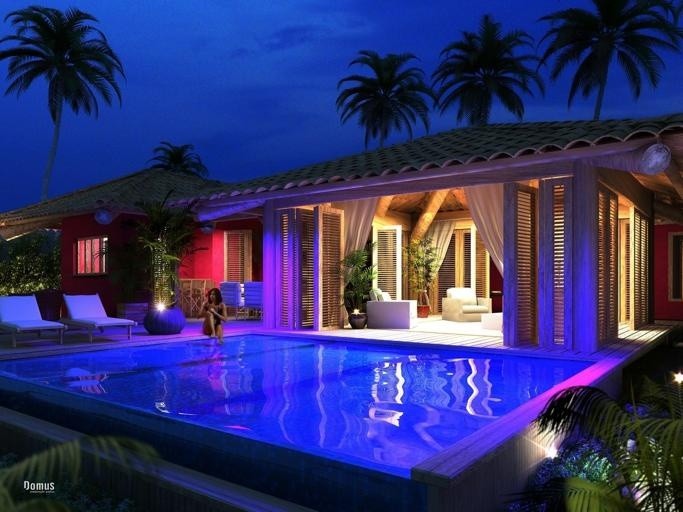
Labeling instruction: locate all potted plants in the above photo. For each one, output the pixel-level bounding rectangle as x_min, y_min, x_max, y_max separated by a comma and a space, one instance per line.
397, 235, 444, 318
89, 187, 213, 335
334, 240, 382, 329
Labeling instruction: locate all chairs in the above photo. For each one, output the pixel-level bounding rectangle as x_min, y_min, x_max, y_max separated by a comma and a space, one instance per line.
218, 281, 263, 321
366, 288, 418, 329
441, 287, 493, 323
0, 291, 137, 348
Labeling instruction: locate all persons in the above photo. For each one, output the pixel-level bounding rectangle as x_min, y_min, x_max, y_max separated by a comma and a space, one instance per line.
197, 287, 227, 343
